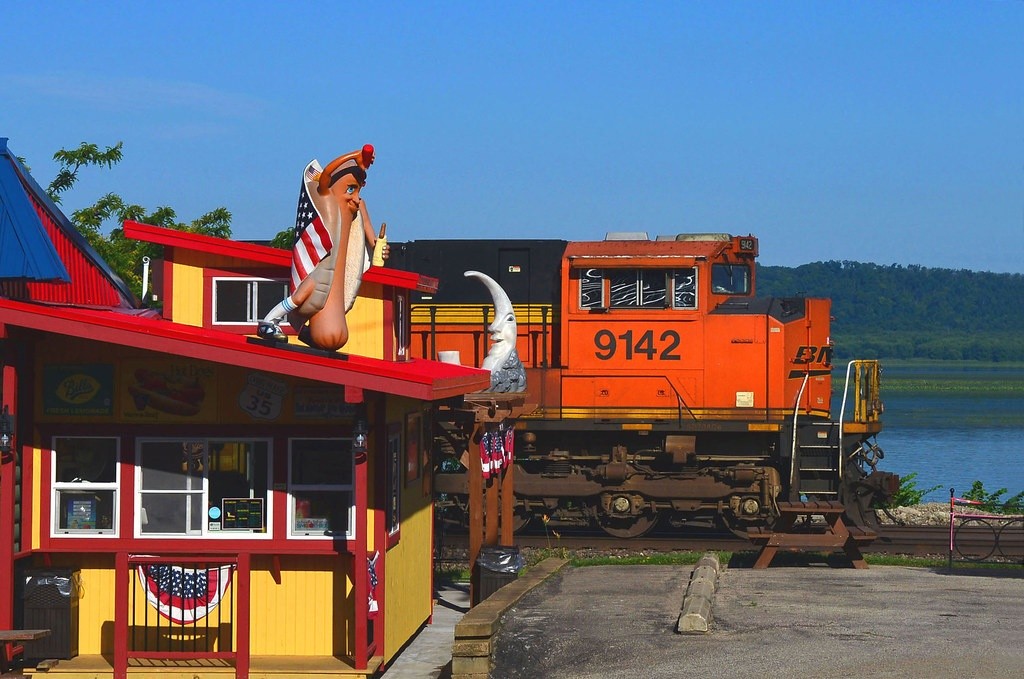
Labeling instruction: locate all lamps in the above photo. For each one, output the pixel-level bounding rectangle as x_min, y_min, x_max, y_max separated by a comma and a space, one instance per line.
0, 404, 14, 450
351, 415, 369, 454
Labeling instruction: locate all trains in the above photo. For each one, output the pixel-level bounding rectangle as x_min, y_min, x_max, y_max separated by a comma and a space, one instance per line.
228, 231, 902, 548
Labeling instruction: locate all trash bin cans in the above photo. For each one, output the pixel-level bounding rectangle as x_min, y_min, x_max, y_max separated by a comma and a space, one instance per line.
476, 544, 520, 604
19, 566, 81, 660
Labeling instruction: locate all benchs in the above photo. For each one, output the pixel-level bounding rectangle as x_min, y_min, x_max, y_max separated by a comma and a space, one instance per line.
0, 629, 51, 640
